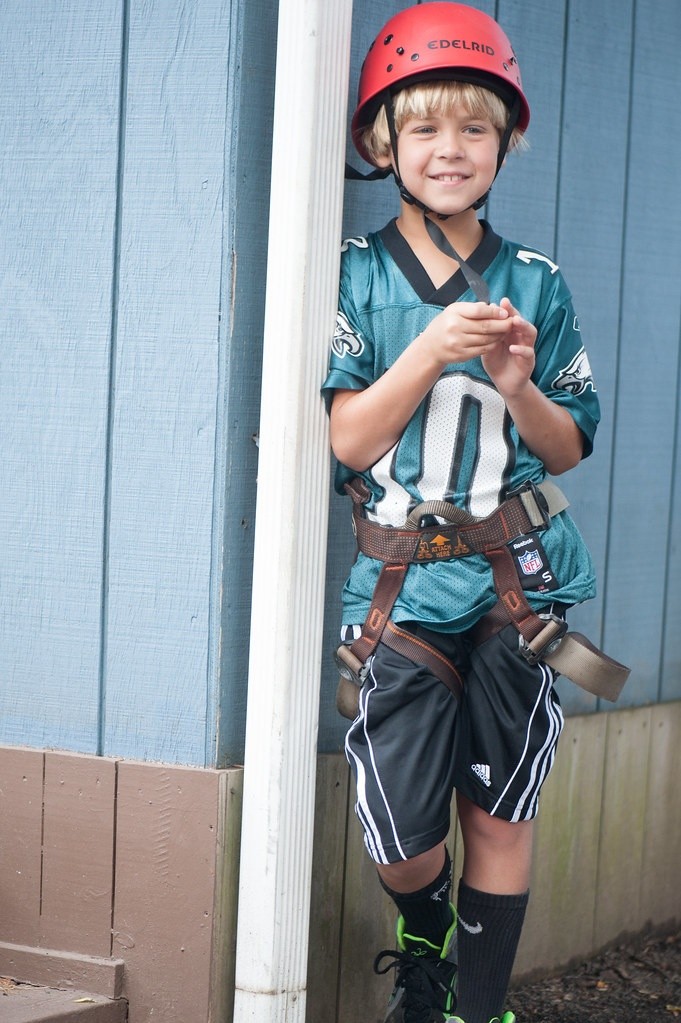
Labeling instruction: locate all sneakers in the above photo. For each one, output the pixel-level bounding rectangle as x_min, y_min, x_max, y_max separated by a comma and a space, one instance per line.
372, 899, 458, 1023
446, 1011, 517, 1023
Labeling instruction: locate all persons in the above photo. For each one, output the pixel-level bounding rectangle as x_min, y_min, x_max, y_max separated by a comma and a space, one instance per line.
333, 1, 634, 1023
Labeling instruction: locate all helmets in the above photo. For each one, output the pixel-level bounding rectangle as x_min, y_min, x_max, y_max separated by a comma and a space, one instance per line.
353, 1, 531, 168
